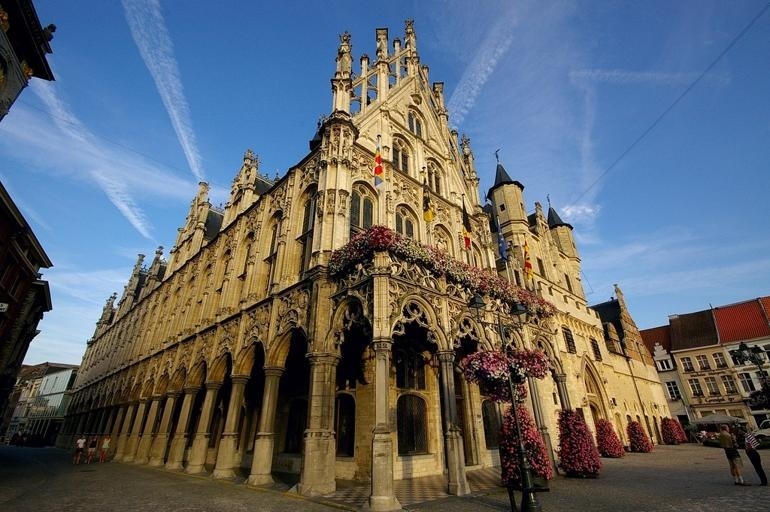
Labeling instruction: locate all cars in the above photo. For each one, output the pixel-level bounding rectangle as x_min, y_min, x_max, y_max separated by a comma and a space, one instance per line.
750, 420, 770, 448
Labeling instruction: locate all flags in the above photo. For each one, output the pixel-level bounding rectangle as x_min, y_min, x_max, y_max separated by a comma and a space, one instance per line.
462, 198, 472, 250
374, 139, 385, 190
496, 217, 508, 259
524, 234, 533, 280
423, 173, 434, 223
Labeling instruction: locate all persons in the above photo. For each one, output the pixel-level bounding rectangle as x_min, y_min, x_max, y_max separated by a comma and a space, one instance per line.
85, 434, 99, 464
720, 424, 752, 487
98, 432, 112, 464
738, 429, 768, 486
73, 434, 86, 464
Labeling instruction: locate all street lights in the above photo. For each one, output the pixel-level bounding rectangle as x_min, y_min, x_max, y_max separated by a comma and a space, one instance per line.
467, 292, 541, 512
